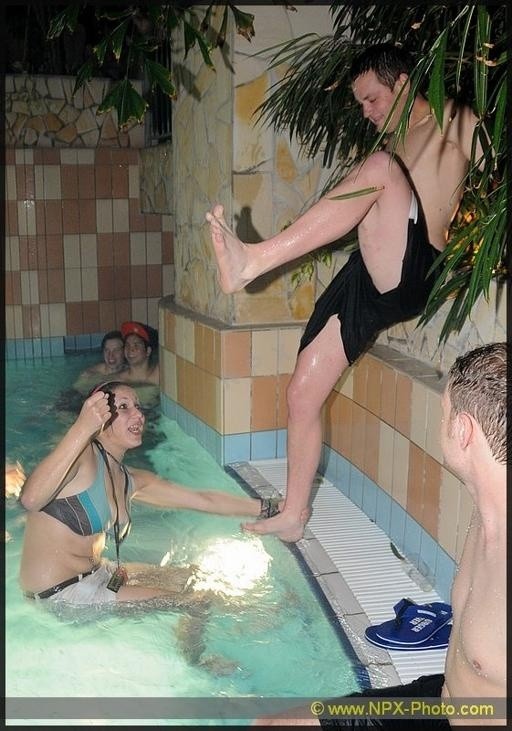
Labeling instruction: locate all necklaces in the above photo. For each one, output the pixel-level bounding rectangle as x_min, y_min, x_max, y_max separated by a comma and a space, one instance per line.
102, 449, 123, 465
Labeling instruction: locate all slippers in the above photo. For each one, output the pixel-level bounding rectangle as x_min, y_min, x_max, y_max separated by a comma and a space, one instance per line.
364, 598, 453, 651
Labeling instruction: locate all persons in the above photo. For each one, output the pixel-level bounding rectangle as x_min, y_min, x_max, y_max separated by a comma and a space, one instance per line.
18, 378, 311, 677
5, 460, 25, 498
252, 342, 511, 725
74, 332, 125, 397
204, 43, 492, 543
110, 320, 159, 410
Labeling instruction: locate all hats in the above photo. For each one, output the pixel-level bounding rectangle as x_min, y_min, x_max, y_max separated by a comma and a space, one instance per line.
120, 321, 149, 342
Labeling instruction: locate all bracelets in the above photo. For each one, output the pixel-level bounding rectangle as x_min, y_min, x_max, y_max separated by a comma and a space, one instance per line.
261, 500, 280, 517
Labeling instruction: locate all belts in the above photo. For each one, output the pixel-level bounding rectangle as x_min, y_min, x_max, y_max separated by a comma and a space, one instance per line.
24, 563, 101, 599
